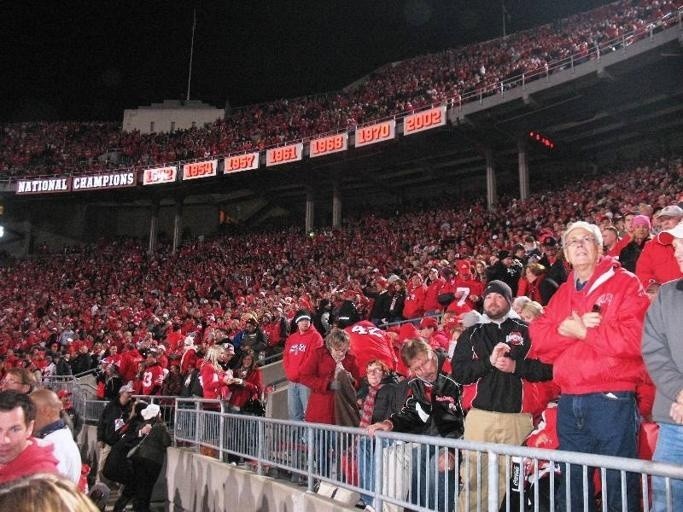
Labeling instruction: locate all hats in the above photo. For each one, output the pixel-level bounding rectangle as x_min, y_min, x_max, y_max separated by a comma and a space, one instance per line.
657, 223, 683, 246
428, 268, 438, 273
542, 237, 557, 247
295, 310, 312, 323
418, 318, 438, 330
220, 343, 235, 355
57, 389, 74, 399
245, 318, 258, 326
482, 281, 513, 306
656, 206, 683, 220
119, 385, 135, 393
141, 404, 163, 420
499, 250, 513, 261
456, 261, 471, 275
376, 279, 386, 288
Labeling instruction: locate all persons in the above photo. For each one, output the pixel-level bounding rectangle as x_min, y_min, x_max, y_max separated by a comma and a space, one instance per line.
368, 337, 464, 512
451, 281, 552, 512
199, 221, 283, 362
529, 222, 648, 511
0, 391, 58, 482
134, 404, 171, 512
635, 204, 682, 292
600, 223, 619, 262
58, 388, 84, 444
28, 388, 81, 488
439, 220, 569, 309
200, 344, 233, 412
0, 236, 198, 403
230, 347, 264, 469
640, 216, 682, 509
297, 329, 361, 491
283, 313, 323, 421
281, 220, 452, 336
342, 159, 683, 218
614, 221, 653, 270
355, 358, 398, 508
58, 391, 78, 431
102, 399, 151, 512
93, 382, 135, 483
0, 1, 682, 185
0, 472, 100, 512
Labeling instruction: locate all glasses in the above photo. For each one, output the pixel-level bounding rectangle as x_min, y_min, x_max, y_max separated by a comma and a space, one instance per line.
410, 353, 438, 376
564, 236, 597, 246
365, 367, 384, 374
1, 378, 27, 386
